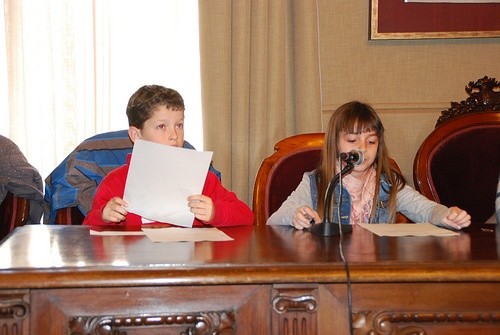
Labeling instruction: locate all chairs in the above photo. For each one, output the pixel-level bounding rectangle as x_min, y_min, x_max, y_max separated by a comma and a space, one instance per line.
413, 76, 500, 223
55, 130, 221, 225
252, 133, 412, 225
0, 134, 31, 241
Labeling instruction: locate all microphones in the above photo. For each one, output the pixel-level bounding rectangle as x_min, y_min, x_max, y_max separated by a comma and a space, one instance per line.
340, 149, 364, 166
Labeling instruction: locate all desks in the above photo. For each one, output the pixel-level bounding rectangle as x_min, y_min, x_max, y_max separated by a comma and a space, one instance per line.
0, 224, 500, 335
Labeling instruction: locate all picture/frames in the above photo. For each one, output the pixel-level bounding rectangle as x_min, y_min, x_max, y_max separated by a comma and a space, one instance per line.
370, 0, 500, 40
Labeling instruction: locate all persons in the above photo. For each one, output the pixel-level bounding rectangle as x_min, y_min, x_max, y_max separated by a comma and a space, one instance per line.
266, 100, 472, 229
82, 85, 256, 231
